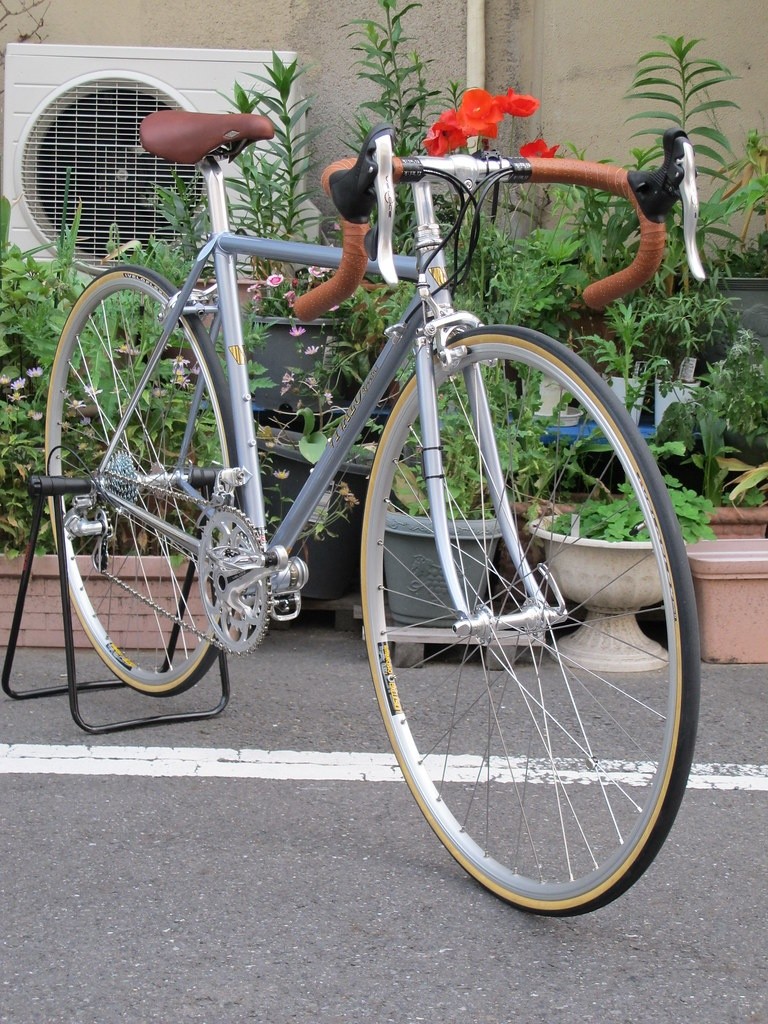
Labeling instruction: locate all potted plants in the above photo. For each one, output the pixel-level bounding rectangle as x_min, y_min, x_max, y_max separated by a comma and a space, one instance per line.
3, 0, 768, 676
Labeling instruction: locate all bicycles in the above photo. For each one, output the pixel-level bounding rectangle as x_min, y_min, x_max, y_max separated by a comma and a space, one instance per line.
41, 108, 708, 918
